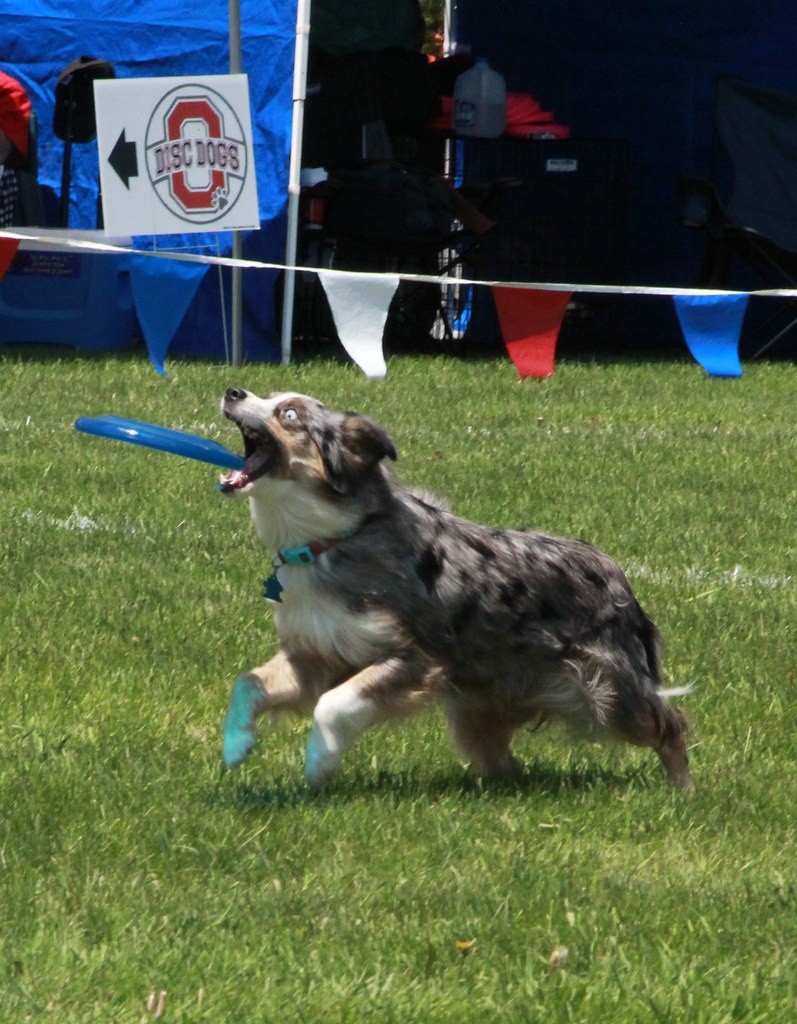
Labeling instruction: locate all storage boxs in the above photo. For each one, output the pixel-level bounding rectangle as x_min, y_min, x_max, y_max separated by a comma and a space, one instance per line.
0, 227, 134, 351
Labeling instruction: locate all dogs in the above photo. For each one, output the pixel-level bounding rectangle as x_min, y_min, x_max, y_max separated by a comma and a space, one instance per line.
217, 387, 699, 799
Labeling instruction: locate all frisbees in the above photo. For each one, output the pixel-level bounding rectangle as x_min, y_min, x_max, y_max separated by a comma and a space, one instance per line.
74, 413, 247, 472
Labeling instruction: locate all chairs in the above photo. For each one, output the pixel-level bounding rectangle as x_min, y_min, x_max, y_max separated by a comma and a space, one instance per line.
306, 48, 502, 356
678, 77, 797, 362
